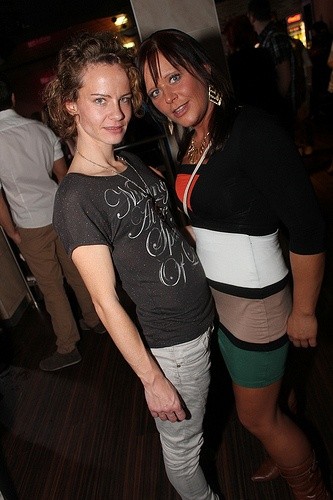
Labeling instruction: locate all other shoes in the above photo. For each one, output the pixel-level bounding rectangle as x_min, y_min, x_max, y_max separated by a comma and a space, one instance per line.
39, 347, 81, 372
80, 317, 107, 334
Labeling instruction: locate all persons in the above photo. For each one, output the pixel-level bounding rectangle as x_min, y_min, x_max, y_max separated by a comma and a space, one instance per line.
53, 34, 224, 499
0, 81, 107, 372
137, 0, 333, 500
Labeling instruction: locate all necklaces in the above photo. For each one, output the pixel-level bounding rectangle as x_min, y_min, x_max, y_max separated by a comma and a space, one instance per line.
186, 131, 210, 163
76, 147, 155, 199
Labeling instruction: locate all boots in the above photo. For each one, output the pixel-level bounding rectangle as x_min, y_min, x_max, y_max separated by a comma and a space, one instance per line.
249, 456, 330, 500
